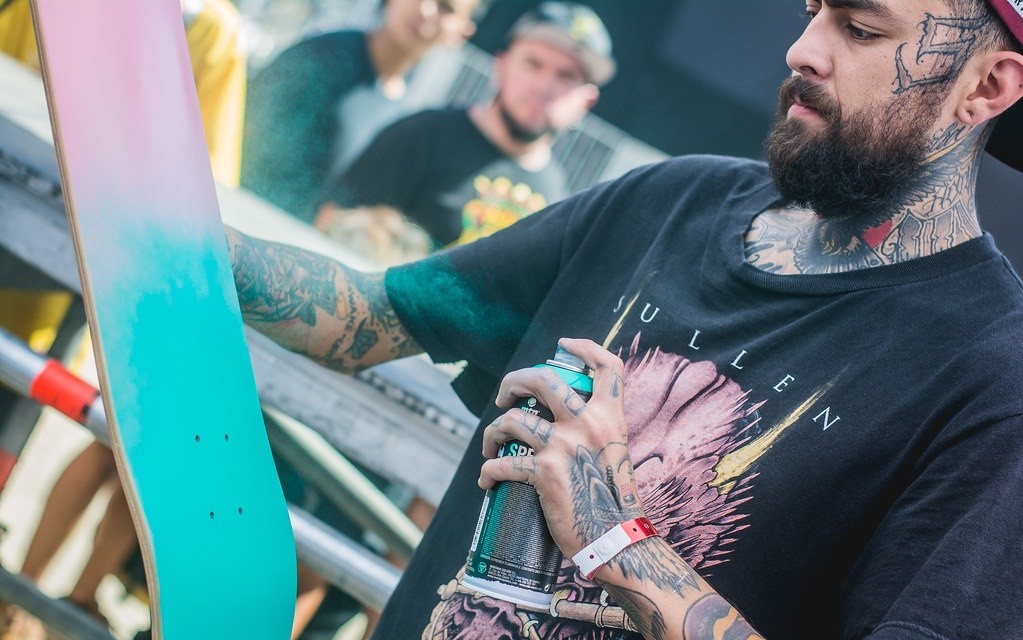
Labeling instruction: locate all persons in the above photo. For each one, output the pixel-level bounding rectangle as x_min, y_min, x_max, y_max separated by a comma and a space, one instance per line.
291, 0, 619, 640
2, 0, 490, 640
223, 0, 1020, 639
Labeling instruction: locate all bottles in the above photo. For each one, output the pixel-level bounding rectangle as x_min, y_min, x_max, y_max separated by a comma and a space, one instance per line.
460, 347, 593, 610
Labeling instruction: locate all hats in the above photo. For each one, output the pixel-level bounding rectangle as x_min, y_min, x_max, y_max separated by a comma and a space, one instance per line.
989, 0, 1023, 46
506, 2, 616, 85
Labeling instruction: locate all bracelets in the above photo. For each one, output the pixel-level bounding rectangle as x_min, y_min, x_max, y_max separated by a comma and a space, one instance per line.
571, 516, 663, 582
